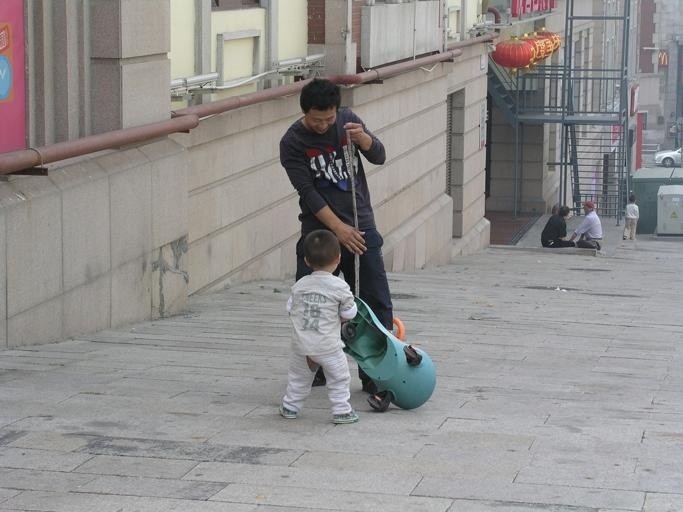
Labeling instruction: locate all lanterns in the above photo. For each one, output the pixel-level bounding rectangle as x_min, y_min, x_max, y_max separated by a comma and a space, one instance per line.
528, 32, 554, 66
537, 28, 561, 52
491, 35, 535, 74
518, 34, 545, 69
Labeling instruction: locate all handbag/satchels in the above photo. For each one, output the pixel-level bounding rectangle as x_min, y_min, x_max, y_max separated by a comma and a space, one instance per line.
575, 240, 596, 248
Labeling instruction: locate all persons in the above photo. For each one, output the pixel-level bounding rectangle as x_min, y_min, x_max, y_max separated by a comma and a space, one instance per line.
568, 201, 602, 250
541, 205, 576, 248
279, 76, 393, 397
279, 229, 359, 424
622, 195, 639, 241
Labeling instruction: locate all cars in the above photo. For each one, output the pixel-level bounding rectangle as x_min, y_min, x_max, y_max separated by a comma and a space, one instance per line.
654, 145, 682, 168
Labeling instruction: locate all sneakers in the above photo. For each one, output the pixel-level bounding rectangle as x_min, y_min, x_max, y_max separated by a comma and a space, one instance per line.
332, 408, 359, 424
279, 405, 297, 420
311, 367, 326, 387
361, 379, 377, 394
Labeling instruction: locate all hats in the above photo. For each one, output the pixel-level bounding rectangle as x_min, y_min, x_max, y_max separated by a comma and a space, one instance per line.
581, 200, 594, 209
